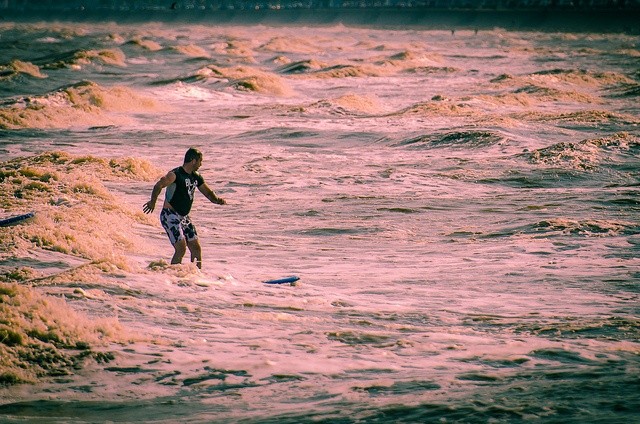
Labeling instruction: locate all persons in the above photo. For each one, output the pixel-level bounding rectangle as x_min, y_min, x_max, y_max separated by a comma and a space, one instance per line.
143, 146, 226, 269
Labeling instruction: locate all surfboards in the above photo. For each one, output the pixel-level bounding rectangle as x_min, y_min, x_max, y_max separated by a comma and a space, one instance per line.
195, 276, 301, 287
